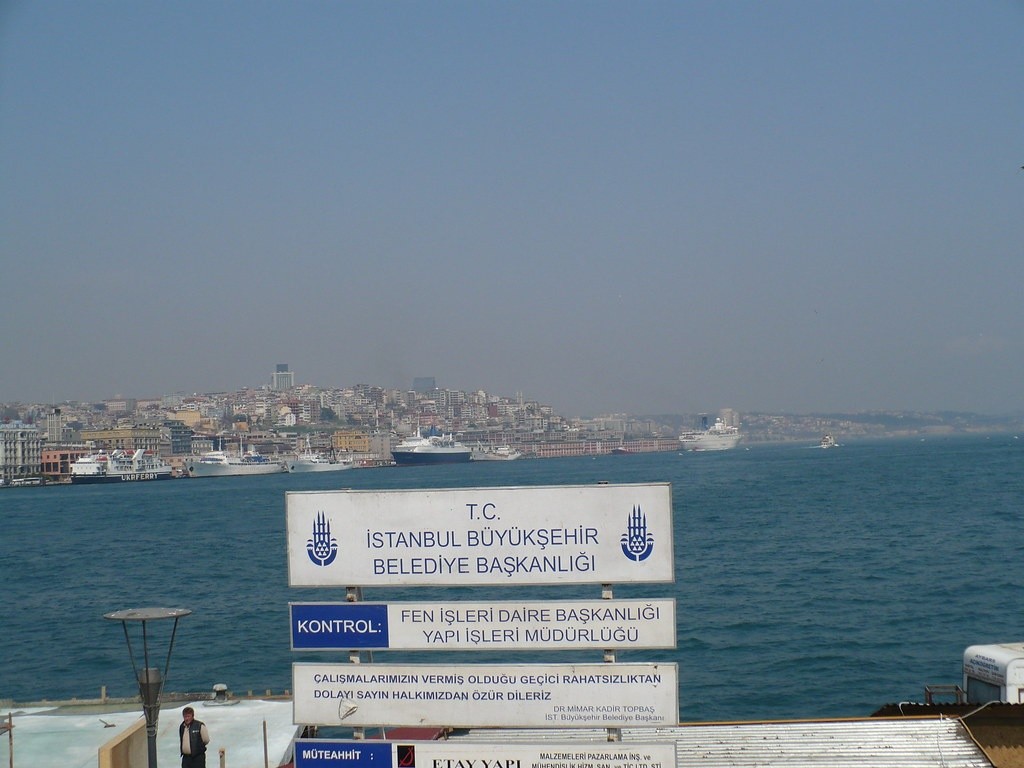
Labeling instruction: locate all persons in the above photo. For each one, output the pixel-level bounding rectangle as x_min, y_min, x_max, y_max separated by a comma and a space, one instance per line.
179, 707, 210, 768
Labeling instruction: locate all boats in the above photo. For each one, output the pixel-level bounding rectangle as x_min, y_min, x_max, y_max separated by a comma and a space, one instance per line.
287, 435, 355, 474
611, 445, 629, 454
820, 434, 835, 449
182, 450, 286, 477
678, 417, 744, 449
69, 448, 182, 484
472, 439, 522, 461
390, 432, 472, 464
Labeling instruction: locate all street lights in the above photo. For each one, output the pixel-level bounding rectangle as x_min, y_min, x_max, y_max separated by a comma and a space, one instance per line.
103, 606, 194, 768
